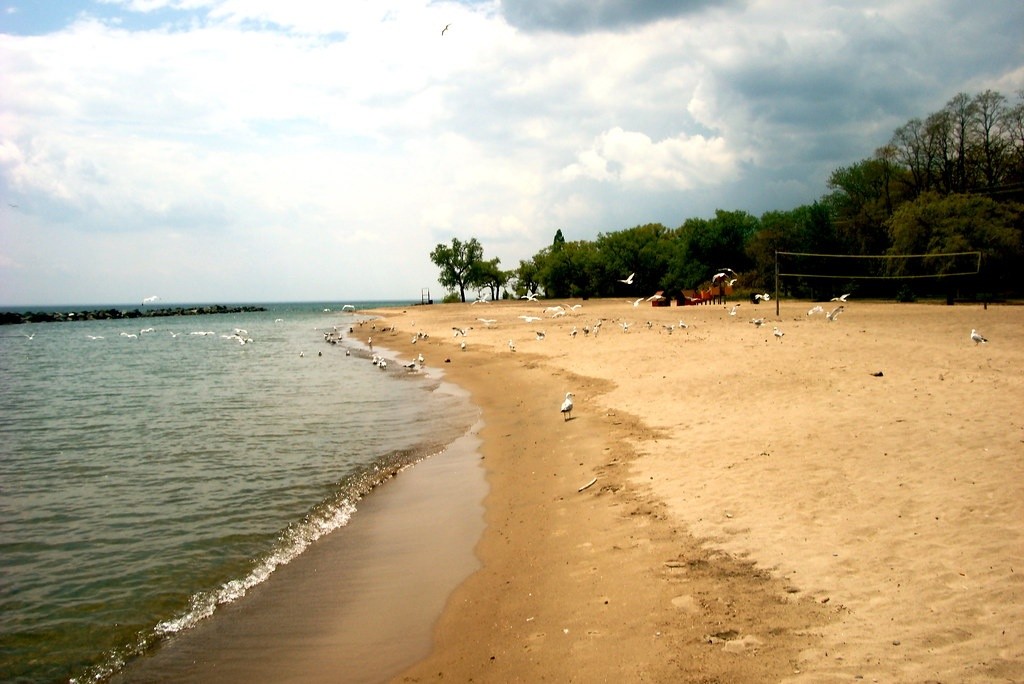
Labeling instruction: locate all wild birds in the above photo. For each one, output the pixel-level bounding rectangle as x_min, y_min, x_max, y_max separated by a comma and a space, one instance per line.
23, 295, 283, 347
560, 392, 575, 421
298, 266, 850, 372
441, 24, 450, 35
970, 328, 988, 347
